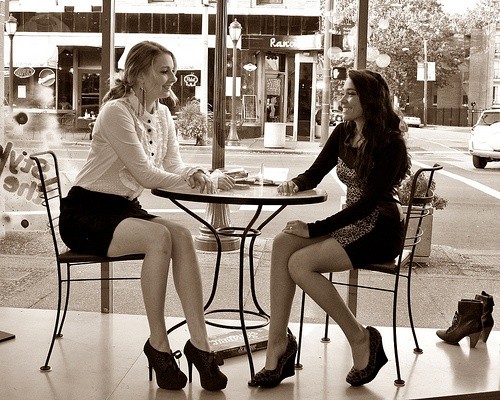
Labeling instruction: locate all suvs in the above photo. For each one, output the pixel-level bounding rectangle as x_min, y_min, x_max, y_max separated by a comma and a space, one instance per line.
329, 108, 345, 125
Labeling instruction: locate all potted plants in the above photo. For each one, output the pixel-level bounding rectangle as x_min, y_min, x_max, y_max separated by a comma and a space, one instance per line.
400, 170, 448, 257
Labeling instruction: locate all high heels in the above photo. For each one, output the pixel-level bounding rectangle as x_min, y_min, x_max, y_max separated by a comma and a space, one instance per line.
251, 334, 297, 388
183, 339, 228, 392
345, 325, 389, 387
144, 338, 188, 391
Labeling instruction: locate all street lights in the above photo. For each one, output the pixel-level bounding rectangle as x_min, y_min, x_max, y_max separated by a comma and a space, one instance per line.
228, 18, 243, 145
5, 16, 19, 114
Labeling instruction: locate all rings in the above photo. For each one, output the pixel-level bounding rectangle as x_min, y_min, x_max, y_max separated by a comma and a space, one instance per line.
289, 226, 293, 230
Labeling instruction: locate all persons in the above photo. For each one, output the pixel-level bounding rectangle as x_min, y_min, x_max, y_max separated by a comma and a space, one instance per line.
247, 70, 412, 386
59, 42, 236, 391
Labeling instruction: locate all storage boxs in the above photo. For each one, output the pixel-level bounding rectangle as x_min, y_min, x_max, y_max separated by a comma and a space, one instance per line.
204, 325, 269, 358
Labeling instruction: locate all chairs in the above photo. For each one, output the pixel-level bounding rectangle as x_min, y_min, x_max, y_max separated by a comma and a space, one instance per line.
295, 163, 443, 386
29, 152, 148, 372
60, 114, 76, 139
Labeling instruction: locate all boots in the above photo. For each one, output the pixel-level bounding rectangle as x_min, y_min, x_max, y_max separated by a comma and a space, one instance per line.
474, 291, 495, 343
435, 299, 484, 348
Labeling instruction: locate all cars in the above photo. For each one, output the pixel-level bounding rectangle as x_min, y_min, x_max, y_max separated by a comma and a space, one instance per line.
470, 105, 500, 169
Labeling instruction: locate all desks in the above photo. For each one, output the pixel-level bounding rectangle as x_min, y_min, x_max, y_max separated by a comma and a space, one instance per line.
151, 180, 327, 380
78, 117, 96, 139
